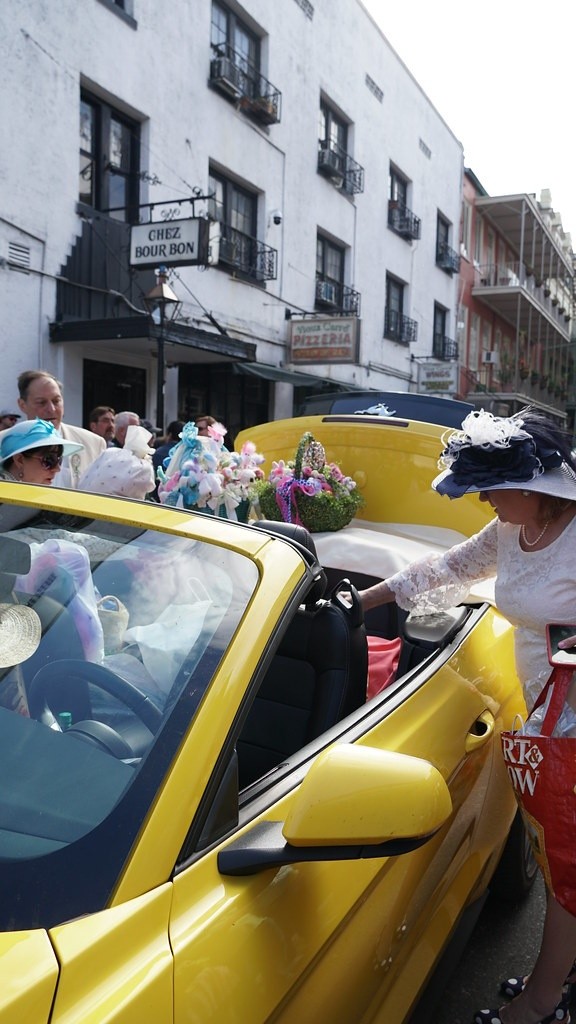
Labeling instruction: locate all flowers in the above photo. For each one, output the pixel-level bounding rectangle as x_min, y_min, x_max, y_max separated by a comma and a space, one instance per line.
437, 404, 553, 471
520, 359, 530, 370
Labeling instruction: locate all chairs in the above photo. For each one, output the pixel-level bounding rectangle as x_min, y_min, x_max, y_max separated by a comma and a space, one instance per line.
159, 523, 369, 793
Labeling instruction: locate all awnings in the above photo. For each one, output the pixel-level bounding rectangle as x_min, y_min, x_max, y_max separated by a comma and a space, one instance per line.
234, 363, 367, 393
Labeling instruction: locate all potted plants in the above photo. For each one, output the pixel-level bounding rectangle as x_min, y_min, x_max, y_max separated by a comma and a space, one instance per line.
526, 266, 572, 322
496, 353, 518, 393
531, 370, 566, 401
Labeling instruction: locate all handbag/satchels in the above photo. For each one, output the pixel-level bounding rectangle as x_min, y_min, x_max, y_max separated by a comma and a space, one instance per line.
96, 595, 129, 654
13, 539, 104, 665
500, 665, 576, 918
123, 576, 212, 695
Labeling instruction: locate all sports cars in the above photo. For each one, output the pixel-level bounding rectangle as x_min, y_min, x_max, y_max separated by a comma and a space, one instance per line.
0, 391, 539, 1024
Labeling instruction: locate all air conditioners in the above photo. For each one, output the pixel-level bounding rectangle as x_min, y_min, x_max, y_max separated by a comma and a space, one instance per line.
439, 252, 452, 267
399, 217, 420, 239
211, 57, 240, 96
317, 282, 335, 306
480, 350, 497, 364
319, 150, 340, 174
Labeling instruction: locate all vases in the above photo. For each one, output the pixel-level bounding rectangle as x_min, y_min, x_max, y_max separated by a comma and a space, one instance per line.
521, 369, 529, 379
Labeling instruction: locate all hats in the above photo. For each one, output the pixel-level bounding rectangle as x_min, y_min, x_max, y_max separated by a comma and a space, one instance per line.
0, 416, 84, 465
0, 407, 21, 418
431, 405, 576, 500
0, 602, 41, 668
140, 420, 162, 432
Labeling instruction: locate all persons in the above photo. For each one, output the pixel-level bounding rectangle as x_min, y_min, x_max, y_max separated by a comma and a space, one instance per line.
0, 410, 22, 431
18, 370, 107, 490
0, 421, 82, 483
113, 412, 140, 448
89, 407, 116, 448
142, 416, 218, 502
298, 410, 576, 1024
86, 545, 233, 715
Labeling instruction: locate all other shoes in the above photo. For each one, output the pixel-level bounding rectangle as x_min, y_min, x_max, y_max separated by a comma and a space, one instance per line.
473, 1000, 570, 1024
497, 976, 571, 1004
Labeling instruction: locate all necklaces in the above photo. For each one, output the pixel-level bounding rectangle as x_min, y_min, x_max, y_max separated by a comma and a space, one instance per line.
523, 522, 548, 546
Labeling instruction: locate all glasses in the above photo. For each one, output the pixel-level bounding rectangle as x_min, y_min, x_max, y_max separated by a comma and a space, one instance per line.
30, 452, 62, 470
195, 426, 207, 431
7, 417, 16, 422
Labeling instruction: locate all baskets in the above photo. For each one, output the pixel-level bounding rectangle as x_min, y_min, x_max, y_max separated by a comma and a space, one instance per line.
157, 436, 250, 523
259, 432, 358, 533
